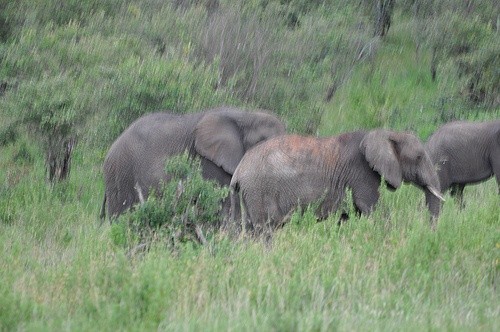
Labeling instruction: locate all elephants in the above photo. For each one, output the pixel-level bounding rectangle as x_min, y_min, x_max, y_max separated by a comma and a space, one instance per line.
227, 129, 446, 240
425, 120, 500, 231
97, 109, 286, 233
